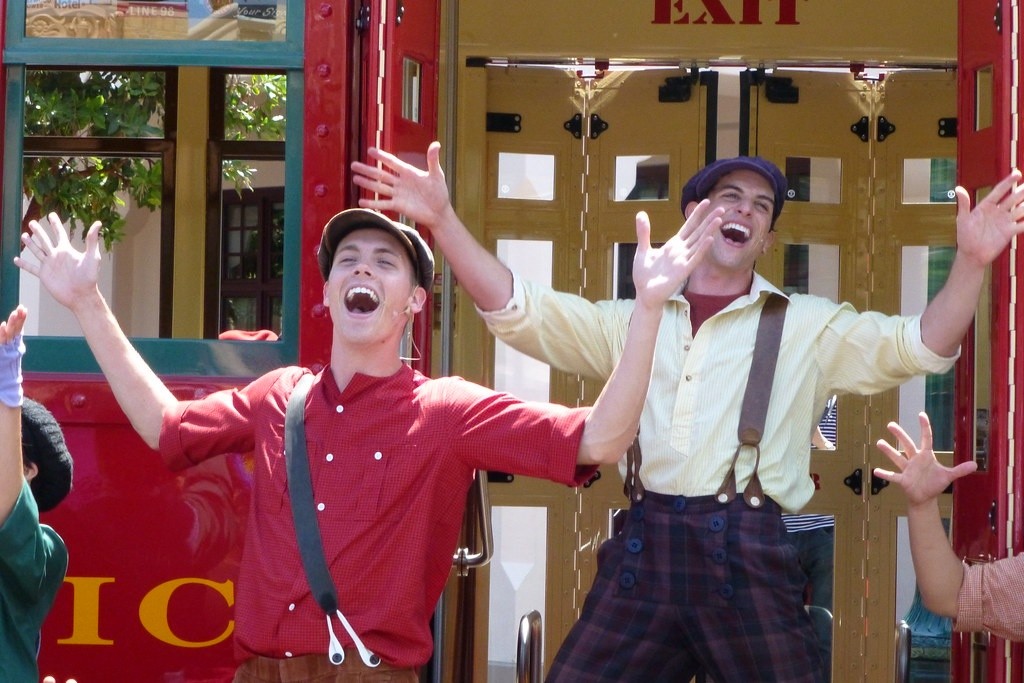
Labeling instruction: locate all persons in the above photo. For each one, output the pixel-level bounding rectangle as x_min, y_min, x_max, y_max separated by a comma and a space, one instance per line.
0, 303, 73, 683
14, 198, 724, 683
350, 142, 1024, 683
873, 410, 1024, 643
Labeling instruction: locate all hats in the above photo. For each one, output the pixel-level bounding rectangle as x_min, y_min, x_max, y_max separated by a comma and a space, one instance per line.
21, 394, 74, 514
681, 155, 788, 232
317, 207, 436, 296
